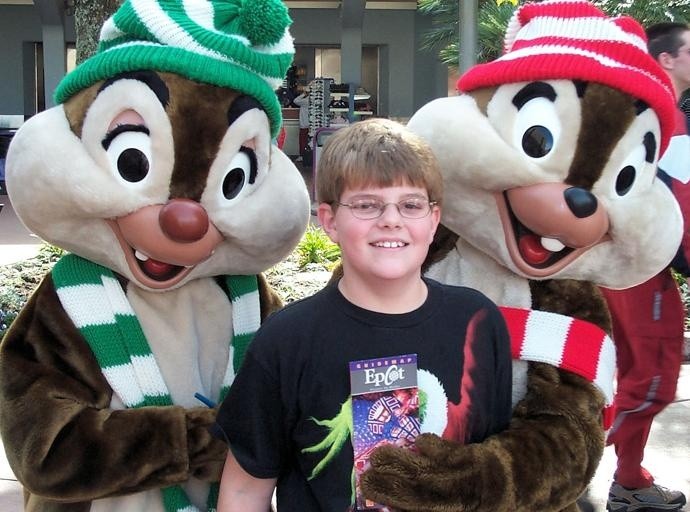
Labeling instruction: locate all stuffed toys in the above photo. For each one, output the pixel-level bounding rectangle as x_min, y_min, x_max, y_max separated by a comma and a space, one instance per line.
404, 2, 684, 512
0, 0, 311, 511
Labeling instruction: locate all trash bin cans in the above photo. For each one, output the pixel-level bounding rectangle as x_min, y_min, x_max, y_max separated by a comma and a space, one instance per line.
312, 128, 344, 201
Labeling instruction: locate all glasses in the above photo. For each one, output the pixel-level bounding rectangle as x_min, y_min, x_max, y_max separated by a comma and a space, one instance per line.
334, 199, 436, 219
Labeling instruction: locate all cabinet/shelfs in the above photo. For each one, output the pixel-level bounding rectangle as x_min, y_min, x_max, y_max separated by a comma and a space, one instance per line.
281, 78, 374, 155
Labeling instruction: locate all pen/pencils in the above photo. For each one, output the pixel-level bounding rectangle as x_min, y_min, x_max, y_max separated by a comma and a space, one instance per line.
194, 392, 216, 408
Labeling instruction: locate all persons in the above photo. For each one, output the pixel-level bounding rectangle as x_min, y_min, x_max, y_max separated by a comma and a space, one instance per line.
598, 23, 690, 512
293, 86, 310, 162
217, 120, 510, 511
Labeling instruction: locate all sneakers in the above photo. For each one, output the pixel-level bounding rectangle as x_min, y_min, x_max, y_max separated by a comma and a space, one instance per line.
606, 480, 686, 511
